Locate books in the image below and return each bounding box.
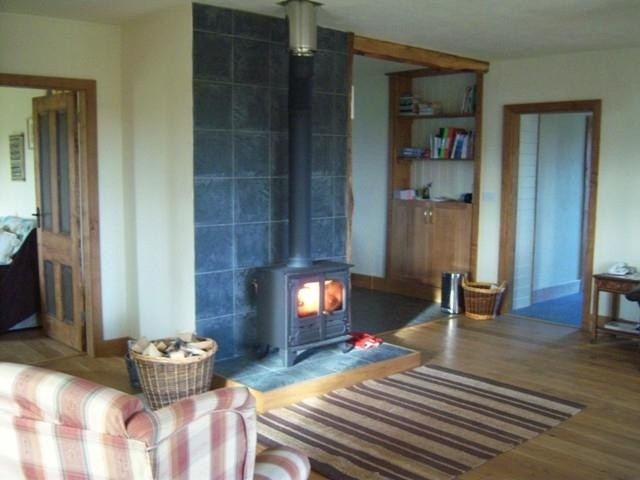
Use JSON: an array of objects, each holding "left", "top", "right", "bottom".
[{"left": 394, "top": 83, "right": 476, "bottom": 205}]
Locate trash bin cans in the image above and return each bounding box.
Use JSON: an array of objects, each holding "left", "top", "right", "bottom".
[{"left": 441, "top": 272, "right": 464, "bottom": 314}]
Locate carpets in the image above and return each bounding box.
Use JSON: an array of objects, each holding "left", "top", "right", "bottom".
[{"left": 257, "top": 364, "right": 587, "bottom": 480}]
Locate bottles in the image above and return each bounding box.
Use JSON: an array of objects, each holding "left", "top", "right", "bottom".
[{"left": 422, "top": 187, "right": 430, "bottom": 199}]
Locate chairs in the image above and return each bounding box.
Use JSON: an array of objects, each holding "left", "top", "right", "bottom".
[
  {"left": 0, "top": 361, "right": 311, "bottom": 480},
  {"left": 0, "top": 216, "right": 42, "bottom": 334}
]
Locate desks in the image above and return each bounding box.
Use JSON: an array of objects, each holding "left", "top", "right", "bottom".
[{"left": 591, "top": 273, "right": 640, "bottom": 343}]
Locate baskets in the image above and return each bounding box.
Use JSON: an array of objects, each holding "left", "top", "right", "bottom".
[
  {"left": 461, "top": 282, "right": 505, "bottom": 320},
  {"left": 129, "top": 337, "right": 217, "bottom": 411}
]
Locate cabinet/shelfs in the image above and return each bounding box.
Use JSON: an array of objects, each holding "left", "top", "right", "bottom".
[{"left": 384, "top": 68, "right": 484, "bottom": 303}]
